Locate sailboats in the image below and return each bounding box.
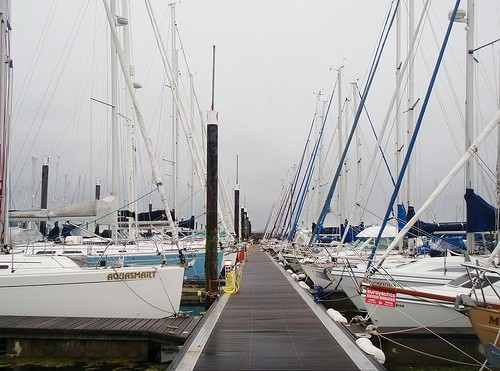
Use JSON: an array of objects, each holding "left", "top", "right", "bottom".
[
  {"left": 260, "top": 0, "right": 500, "bottom": 371},
  {"left": 0, "top": 0, "right": 248, "bottom": 319}
]
